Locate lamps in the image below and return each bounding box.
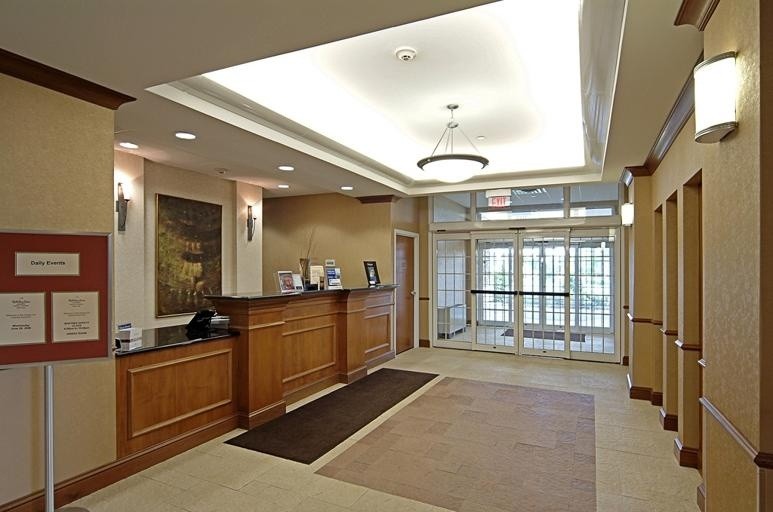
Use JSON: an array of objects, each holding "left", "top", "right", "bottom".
[
  {"left": 412, "top": 100, "right": 491, "bottom": 186},
  {"left": 619, "top": 202, "right": 634, "bottom": 228},
  {"left": 246, "top": 204, "right": 256, "bottom": 241},
  {"left": 691, "top": 49, "right": 737, "bottom": 144},
  {"left": 114, "top": 181, "right": 128, "bottom": 232}
]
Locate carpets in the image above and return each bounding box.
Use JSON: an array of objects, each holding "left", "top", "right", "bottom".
[
  {"left": 312, "top": 375, "right": 598, "bottom": 512},
  {"left": 219, "top": 364, "right": 442, "bottom": 467},
  {"left": 500, "top": 328, "right": 585, "bottom": 343}
]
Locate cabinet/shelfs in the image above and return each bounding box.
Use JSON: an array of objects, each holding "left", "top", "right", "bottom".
[{"left": 438, "top": 304, "right": 466, "bottom": 339}]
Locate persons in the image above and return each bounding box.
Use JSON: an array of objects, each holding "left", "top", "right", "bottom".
[{"left": 175, "top": 236, "right": 206, "bottom": 282}]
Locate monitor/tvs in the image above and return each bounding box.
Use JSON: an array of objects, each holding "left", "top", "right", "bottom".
[{"left": 304, "top": 283, "right": 318, "bottom": 291}]
